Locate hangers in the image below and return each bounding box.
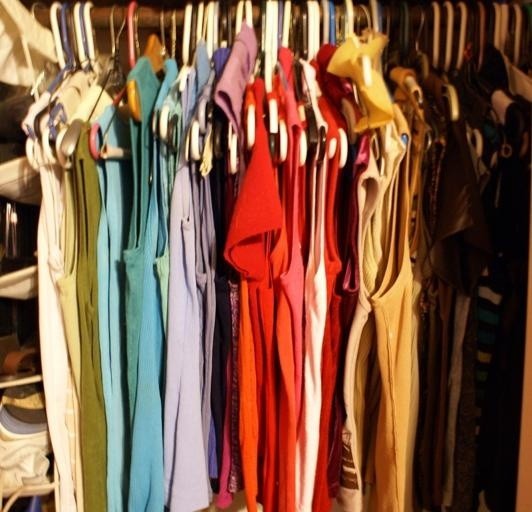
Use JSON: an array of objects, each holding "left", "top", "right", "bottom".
[{"left": 20, "top": 2, "right": 532, "bottom": 174}]
